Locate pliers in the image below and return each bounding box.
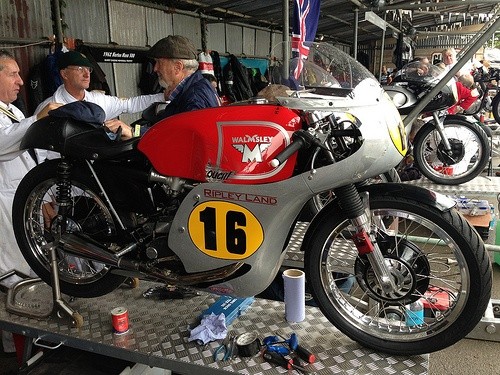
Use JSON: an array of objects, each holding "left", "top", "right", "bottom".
[{"left": 214, "top": 337, "right": 230, "bottom": 361}]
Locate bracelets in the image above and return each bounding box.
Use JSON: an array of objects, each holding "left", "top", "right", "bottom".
[{"left": 131, "top": 124, "right": 140, "bottom": 138}]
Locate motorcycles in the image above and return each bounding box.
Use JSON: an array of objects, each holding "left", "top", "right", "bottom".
[
  {"left": 376, "top": 54, "right": 500, "bottom": 185},
  {"left": 10, "top": 39, "right": 494, "bottom": 357}
]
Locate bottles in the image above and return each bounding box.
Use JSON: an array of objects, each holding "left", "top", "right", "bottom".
[
  {"left": 489, "top": 207, "right": 495, "bottom": 231},
  {"left": 405, "top": 300, "right": 424, "bottom": 326}
]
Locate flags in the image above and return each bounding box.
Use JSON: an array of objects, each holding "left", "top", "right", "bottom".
[{"left": 290, "top": 0, "right": 321, "bottom": 80}]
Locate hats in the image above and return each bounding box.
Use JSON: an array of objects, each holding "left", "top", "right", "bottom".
[
  {"left": 148, "top": 34, "right": 198, "bottom": 60},
  {"left": 56, "top": 51, "right": 94, "bottom": 69},
  {"left": 49, "top": 101, "right": 106, "bottom": 125}
]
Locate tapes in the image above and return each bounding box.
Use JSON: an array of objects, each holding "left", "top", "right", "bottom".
[{"left": 236, "top": 331, "right": 262, "bottom": 358}]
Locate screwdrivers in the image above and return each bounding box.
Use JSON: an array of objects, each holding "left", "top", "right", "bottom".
[
  {"left": 263, "top": 350, "right": 316, "bottom": 374},
  {"left": 275, "top": 331, "right": 316, "bottom": 364}
]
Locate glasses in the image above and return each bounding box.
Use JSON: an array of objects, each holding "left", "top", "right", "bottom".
[{"left": 67, "top": 66, "right": 93, "bottom": 73}]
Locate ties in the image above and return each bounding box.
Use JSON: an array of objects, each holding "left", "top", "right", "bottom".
[{"left": 6, "top": 110, "right": 39, "bottom": 165}]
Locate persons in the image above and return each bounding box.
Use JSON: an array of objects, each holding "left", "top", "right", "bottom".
[
  {"left": 381, "top": 47, "right": 490, "bottom": 176},
  {"left": 0, "top": 35, "right": 223, "bottom": 364}
]
